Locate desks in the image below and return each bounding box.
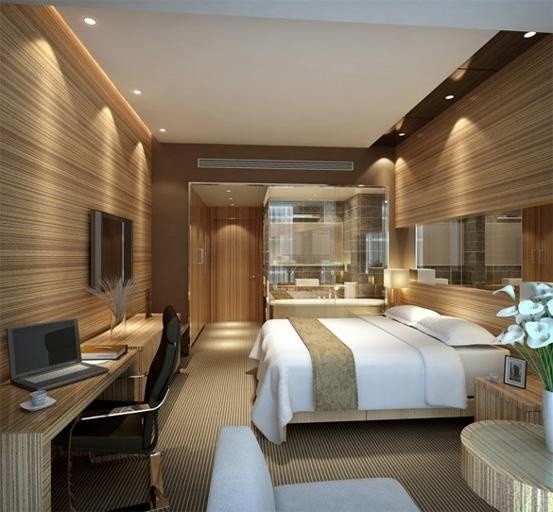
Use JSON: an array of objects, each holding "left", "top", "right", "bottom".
[
  {"left": 1, "top": 348, "right": 140, "bottom": 512},
  {"left": 459, "top": 419, "right": 553, "bottom": 511}
]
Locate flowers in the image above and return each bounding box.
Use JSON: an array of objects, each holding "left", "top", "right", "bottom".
[{"left": 491, "top": 284, "right": 553, "bottom": 393}]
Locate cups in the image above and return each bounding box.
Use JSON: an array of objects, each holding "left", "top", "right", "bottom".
[
  {"left": 30, "top": 389, "right": 48, "bottom": 406},
  {"left": 488, "top": 373, "right": 500, "bottom": 384}
]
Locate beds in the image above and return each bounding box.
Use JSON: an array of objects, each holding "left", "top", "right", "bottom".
[{"left": 247, "top": 314, "right": 512, "bottom": 446}]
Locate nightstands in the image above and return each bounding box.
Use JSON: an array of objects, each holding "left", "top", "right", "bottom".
[{"left": 472, "top": 376, "right": 542, "bottom": 422}]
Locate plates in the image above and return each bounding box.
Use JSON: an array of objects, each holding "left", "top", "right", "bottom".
[{"left": 19, "top": 396, "right": 56, "bottom": 411}]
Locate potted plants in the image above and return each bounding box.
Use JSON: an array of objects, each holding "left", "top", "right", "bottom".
[{"left": 85, "top": 275, "right": 140, "bottom": 339}]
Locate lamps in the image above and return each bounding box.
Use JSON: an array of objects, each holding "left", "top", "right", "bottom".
[
  {"left": 416, "top": 269, "right": 436, "bottom": 287},
  {"left": 384, "top": 268, "right": 409, "bottom": 305}
]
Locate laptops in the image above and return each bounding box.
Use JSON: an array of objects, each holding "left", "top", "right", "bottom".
[{"left": 7, "top": 319, "right": 108, "bottom": 392}]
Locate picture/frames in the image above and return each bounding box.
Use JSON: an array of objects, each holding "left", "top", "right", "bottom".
[{"left": 504, "top": 355, "right": 527, "bottom": 389}]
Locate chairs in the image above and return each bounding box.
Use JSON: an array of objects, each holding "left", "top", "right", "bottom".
[{"left": 54, "top": 304, "right": 182, "bottom": 512}]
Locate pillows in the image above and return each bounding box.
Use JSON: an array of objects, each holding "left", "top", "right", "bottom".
[
  {"left": 383, "top": 304, "right": 441, "bottom": 327},
  {"left": 414, "top": 315, "right": 499, "bottom": 347}
]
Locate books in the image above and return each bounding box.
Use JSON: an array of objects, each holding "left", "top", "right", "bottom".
[{"left": 79, "top": 343, "right": 128, "bottom": 361}]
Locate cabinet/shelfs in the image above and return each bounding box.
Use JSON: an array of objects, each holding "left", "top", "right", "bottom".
[{"left": 80, "top": 313, "right": 182, "bottom": 401}]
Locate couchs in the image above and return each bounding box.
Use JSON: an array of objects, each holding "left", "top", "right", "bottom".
[{"left": 205, "top": 424, "right": 420, "bottom": 511}]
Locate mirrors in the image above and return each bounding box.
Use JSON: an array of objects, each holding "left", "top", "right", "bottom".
[{"left": 415, "top": 208, "right": 523, "bottom": 296}]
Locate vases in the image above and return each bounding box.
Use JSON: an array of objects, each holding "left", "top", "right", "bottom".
[{"left": 542, "top": 389, "right": 553, "bottom": 453}]
